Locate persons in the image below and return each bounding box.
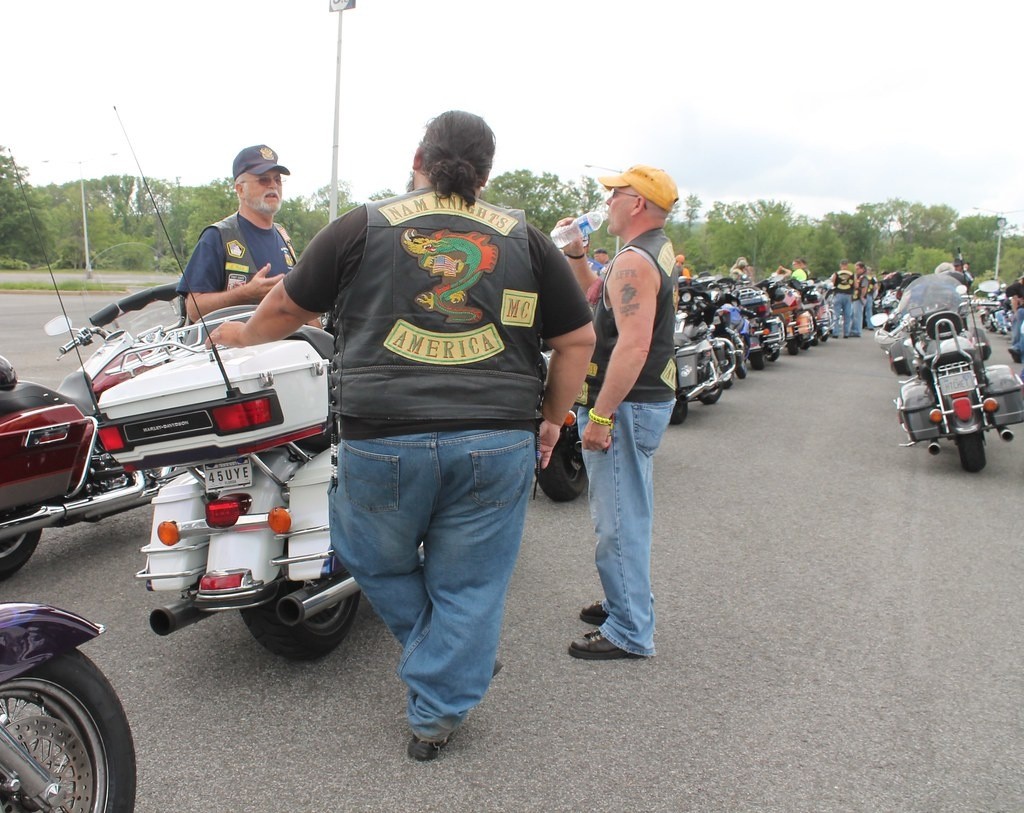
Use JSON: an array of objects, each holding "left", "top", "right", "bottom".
[
  {"left": 555, "top": 164, "right": 679, "bottom": 661},
  {"left": 205, "top": 110, "right": 596, "bottom": 762},
  {"left": 771, "top": 259, "right": 810, "bottom": 281},
  {"left": 593, "top": 249, "right": 611, "bottom": 264},
  {"left": 584, "top": 234, "right": 604, "bottom": 272},
  {"left": 988, "top": 296, "right": 1012, "bottom": 335},
  {"left": 865, "top": 267, "right": 878, "bottom": 331},
  {"left": 954, "top": 258, "right": 974, "bottom": 287},
  {"left": 849, "top": 263, "right": 868, "bottom": 337},
  {"left": 1005, "top": 276, "right": 1024, "bottom": 363},
  {"left": 175, "top": 145, "right": 322, "bottom": 329},
  {"left": 831, "top": 259, "right": 855, "bottom": 338},
  {"left": 729, "top": 256, "right": 753, "bottom": 280},
  {"left": 676, "top": 254, "right": 685, "bottom": 275}
]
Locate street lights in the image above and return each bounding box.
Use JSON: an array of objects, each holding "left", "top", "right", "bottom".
[
  {"left": 583, "top": 162, "right": 619, "bottom": 254},
  {"left": 970, "top": 206, "right": 1024, "bottom": 280}
]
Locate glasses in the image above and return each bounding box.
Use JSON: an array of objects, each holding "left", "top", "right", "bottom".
[
  {"left": 240, "top": 175, "right": 285, "bottom": 186},
  {"left": 612, "top": 189, "right": 648, "bottom": 209}
]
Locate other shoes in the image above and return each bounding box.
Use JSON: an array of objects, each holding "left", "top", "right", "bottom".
[
  {"left": 408, "top": 731, "right": 455, "bottom": 758},
  {"left": 1008, "top": 347, "right": 1021, "bottom": 363},
  {"left": 492, "top": 660, "right": 503, "bottom": 676}
]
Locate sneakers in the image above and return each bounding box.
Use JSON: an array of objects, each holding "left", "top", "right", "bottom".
[
  {"left": 569, "top": 630, "right": 645, "bottom": 659},
  {"left": 579, "top": 600, "right": 610, "bottom": 625}
]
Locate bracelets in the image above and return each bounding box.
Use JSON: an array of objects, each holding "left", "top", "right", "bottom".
[
  {"left": 567, "top": 253, "right": 584, "bottom": 259},
  {"left": 588, "top": 408, "right": 612, "bottom": 426}
]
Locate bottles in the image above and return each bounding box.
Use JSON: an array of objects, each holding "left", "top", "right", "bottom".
[{"left": 551, "top": 209, "right": 608, "bottom": 248}]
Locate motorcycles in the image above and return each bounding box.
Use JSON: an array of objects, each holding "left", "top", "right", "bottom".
[
  {"left": 531, "top": 270, "right": 1012, "bottom": 503},
  {"left": 92, "top": 304, "right": 425, "bottom": 662},
  {"left": 0, "top": 242, "right": 188, "bottom": 586},
  {"left": 870, "top": 272, "right": 1024, "bottom": 474},
  {"left": 0, "top": 599, "right": 139, "bottom": 813}
]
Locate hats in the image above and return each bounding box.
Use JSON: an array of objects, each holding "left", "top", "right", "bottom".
[
  {"left": 841, "top": 259, "right": 848, "bottom": 264},
  {"left": 739, "top": 260, "right": 748, "bottom": 267},
  {"left": 955, "top": 261, "right": 961, "bottom": 265},
  {"left": 233, "top": 145, "right": 290, "bottom": 180},
  {"left": 934, "top": 262, "right": 955, "bottom": 274},
  {"left": 598, "top": 164, "right": 679, "bottom": 212},
  {"left": 594, "top": 248, "right": 607, "bottom": 253}
]
